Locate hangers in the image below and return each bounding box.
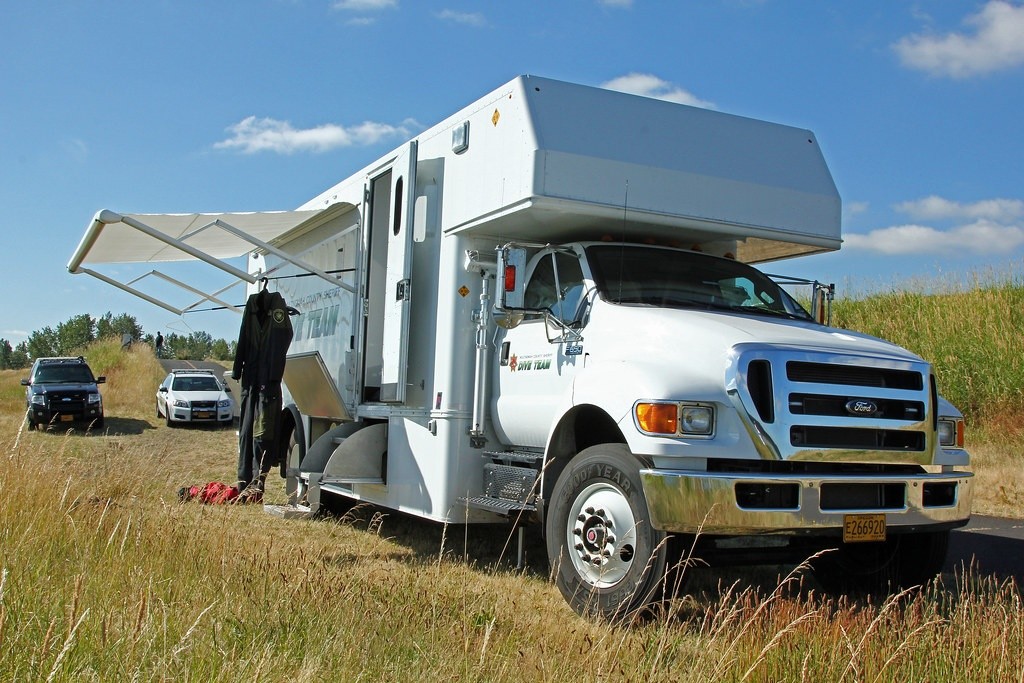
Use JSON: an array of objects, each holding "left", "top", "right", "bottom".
[{"left": 257, "top": 277, "right": 268, "bottom": 293}]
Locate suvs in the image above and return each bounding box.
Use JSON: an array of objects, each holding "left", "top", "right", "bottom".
[{"left": 19, "top": 355, "right": 107, "bottom": 430}]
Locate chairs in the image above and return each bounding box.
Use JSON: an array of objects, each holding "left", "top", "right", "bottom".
[{"left": 175, "top": 381, "right": 191, "bottom": 391}]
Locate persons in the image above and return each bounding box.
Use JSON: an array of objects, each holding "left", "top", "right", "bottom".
[{"left": 155, "top": 331, "right": 163, "bottom": 358}]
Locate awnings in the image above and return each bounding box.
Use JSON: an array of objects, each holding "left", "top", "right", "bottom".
[{"left": 66, "top": 202, "right": 359, "bottom": 316}]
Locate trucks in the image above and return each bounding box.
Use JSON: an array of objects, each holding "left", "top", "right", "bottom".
[{"left": 66, "top": 74, "right": 977, "bottom": 631}]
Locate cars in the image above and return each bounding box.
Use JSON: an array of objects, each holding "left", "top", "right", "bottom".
[{"left": 155, "top": 369, "right": 234, "bottom": 428}]
